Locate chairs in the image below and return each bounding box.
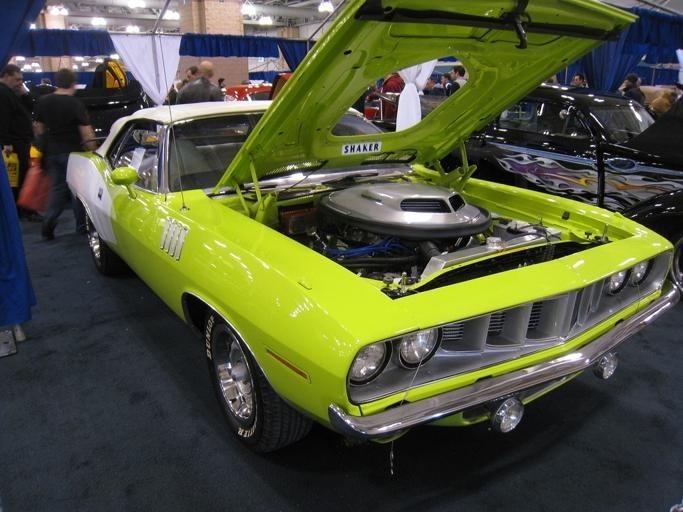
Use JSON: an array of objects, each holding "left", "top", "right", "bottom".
[{"left": 150, "top": 137, "right": 212, "bottom": 189}]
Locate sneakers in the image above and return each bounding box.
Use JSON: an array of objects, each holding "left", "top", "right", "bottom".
[
  {"left": 1, "top": 325, "right": 26, "bottom": 357},
  {"left": 29, "top": 214, "right": 55, "bottom": 239}
]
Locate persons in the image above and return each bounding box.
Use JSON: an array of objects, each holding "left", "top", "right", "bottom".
[
  {"left": 567, "top": 72, "right": 585, "bottom": 89},
  {"left": 617, "top": 72, "right": 646, "bottom": 107},
  {"left": 31, "top": 66, "right": 99, "bottom": 241},
  {"left": 0, "top": 64, "right": 46, "bottom": 224},
  {"left": 364, "top": 64, "right": 469, "bottom": 123},
  {"left": 641, "top": 86, "right": 678, "bottom": 120},
  {"left": 164, "top": 60, "right": 228, "bottom": 107}
]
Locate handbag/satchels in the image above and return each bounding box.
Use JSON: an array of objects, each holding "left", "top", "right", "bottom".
[{"left": 19, "top": 167, "right": 52, "bottom": 212}]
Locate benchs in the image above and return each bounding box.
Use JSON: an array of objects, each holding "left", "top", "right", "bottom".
[{"left": 137, "top": 142, "right": 244, "bottom": 191}]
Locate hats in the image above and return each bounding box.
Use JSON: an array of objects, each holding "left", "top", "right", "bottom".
[{"left": 626, "top": 74, "right": 637, "bottom": 83}]
